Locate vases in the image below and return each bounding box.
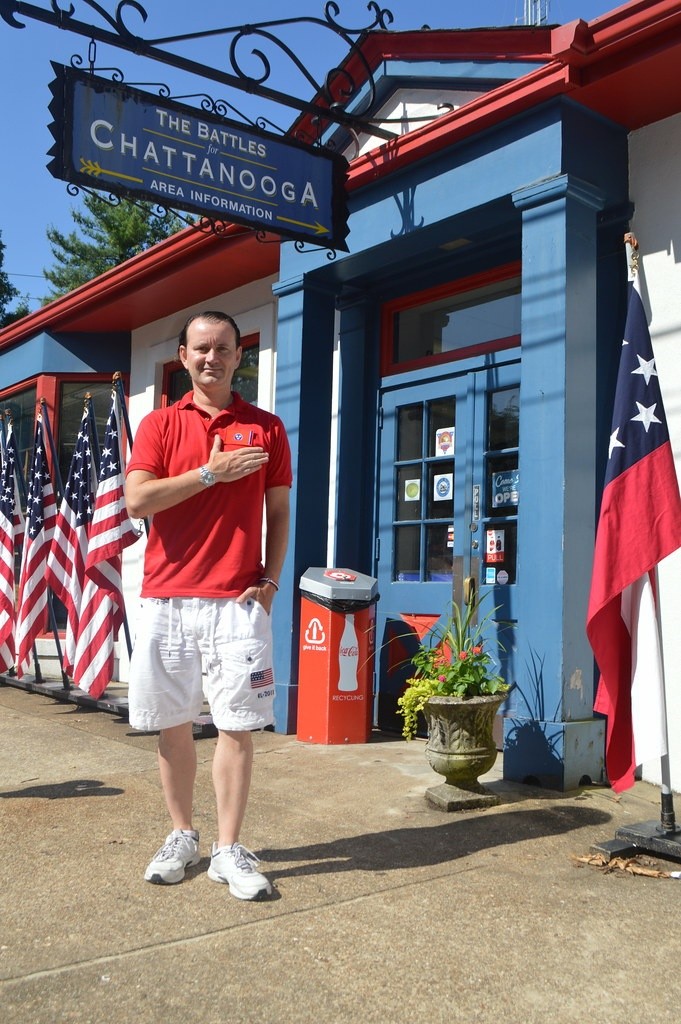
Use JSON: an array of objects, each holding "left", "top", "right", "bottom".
[{"left": 420, "top": 689, "right": 510, "bottom": 814}]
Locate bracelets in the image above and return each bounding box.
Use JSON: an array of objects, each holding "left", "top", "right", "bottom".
[{"left": 261, "top": 578, "right": 279, "bottom": 591}]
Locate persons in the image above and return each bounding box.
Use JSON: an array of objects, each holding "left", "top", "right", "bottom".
[{"left": 124, "top": 313, "right": 293, "bottom": 899}]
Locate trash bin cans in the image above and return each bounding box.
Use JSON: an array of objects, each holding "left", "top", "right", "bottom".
[{"left": 295, "top": 567, "right": 380, "bottom": 746}]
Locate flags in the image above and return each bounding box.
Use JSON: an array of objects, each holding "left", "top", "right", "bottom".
[
  {"left": 44, "top": 402, "right": 98, "bottom": 676},
  {"left": 0, "top": 419, "right": 25, "bottom": 673},
  {"left": 15, "top": 409, "right": 51, "bottom": 679},
  {"left": 587, "top": 268, "right": 681, "bottom": 794},
  {"left": 72, "top": 390, "right": 140, "bottom": 699}
]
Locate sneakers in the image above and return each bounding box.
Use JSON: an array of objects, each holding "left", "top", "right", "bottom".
[
  {"left": 144, "top": 829, "right": 201, "bottom": 885},
  {"left": 207, "top": 841, "right": 272, "bottom": 901}
]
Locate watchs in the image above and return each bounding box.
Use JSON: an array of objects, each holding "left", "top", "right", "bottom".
[{"left": 199, "top": 466, "right": 216, "bottom": 488}]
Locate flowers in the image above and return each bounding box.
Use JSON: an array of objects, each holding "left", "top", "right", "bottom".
[{"left": 350, "top": 588, "right": 521, "bottom": 741}]
